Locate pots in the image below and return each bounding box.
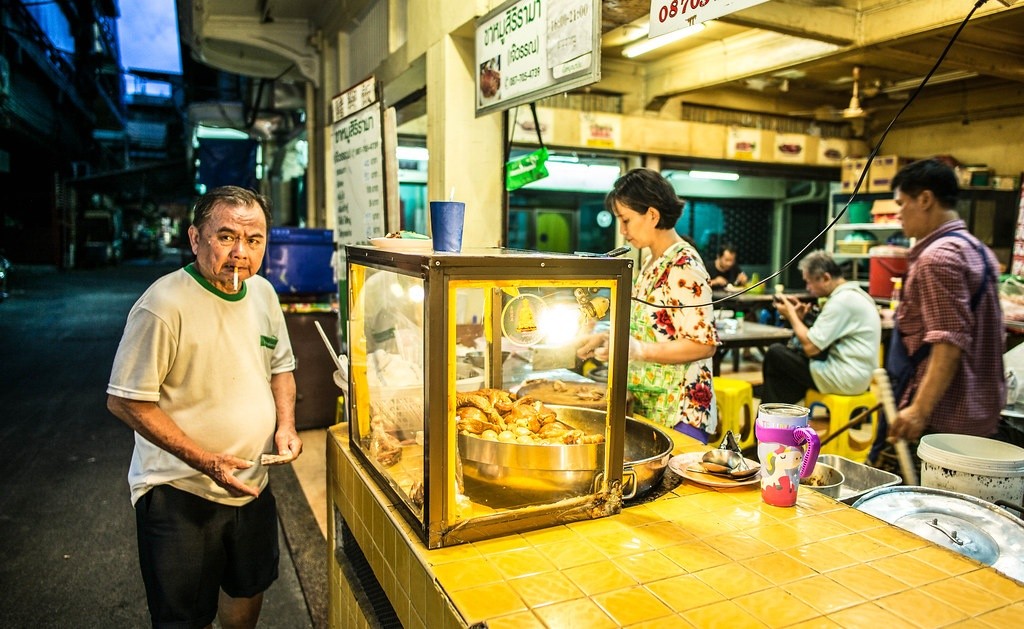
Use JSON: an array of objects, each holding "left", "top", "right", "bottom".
[
  {"left": 851, "top": 485, "right": 1024, "bottom": 583},
  {"left": 456, "top": 403, "right": 675, "bottom": 512}
]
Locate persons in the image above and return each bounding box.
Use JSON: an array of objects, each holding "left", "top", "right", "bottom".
[
  {"left": 575, "top": 168, "right": 722, "bottom": 445},
  {"left": 681, "top": 234, "right": 694, "bottom": 250},
  {"left": 704, "top": 243, "right": 761, "bottom": 364},
  {"left": 108, "top": 185, "right": 303, "bottom": 629},
  {"left": 869, "top": 159, "right": 1007, "bottom": 467},
  {"left": 762, "top": 249, "right": 881, "bottom": 404}
]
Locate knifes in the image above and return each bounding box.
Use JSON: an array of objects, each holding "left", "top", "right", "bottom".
[{"left": 533, "top": 346, "right": 578, "bottom": 371}]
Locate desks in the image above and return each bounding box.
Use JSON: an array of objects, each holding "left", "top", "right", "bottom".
[
  {"left": 712, "top": 285, "right": 820, "bottom": 368},
  {"left": 714, "top": 313, "right": 794, "bottom": 376}
]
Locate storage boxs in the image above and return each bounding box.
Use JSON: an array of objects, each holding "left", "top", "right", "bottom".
[
  {"left": 867, "top": 157, "right": 915, "bottom": 193},
  {"left": 838, "top": 157, "right": 868, "bottom": 194},
  {"left": 871, "top": 198, "right": 903, "bottom": 223}
]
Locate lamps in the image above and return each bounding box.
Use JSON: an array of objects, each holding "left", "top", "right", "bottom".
[{"left": 619, "top": 17, "right": 709, "bottom": 62}]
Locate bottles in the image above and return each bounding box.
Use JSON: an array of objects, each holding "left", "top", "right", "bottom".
[
  {"left": 890, "top": 281, "right": 902, "bottom": 310},
  {"left": 736, "top": 312, "right": 744, "bottom": 329}
]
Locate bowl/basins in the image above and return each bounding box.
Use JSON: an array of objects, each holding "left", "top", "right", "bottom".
[
  {"left": 800, "top": 461, "right": 846, "bottom": 498},
  {"left": 879, "top": 309, "right": 896, "bottom": 322},
  {"left": 465, "top": 351, "right": 511, "bottom": 369},
  {"left": 713, "top": 309, "right": 734, "bottom": 328}
]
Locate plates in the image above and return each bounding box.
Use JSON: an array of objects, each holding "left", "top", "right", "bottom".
[
  {"left": 521, "top": 121, "right": 548, "bottom": 134},
  {"left": 367, "top": 236, "right": 432, "bottom": 247},
  {"left": 734, "top": 140, "right": 843, "bottom": 161},
  {"left": 668, "top": 450, "right": 763, "bottom": 487},
  {"left": 480, "top": 71, "right": 500, "bottom": 105},
  {"left": 724, "top": 287, "right": 744, "bottom": 293}
]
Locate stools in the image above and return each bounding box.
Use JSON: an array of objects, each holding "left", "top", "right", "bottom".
[
  {"left": 712, "top": 376, "right": 754, "bottom": 450},
  {"left": 808, "top": 389, "right": 883, "bottom": 463}
]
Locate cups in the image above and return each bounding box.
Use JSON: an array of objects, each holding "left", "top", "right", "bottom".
[
  {"left": 723, "top": 319, "right": 738, "bottom": 335},
  {"left": 429, "top": 201, "right": 466, "bottom": 253},
  {"left": 754, "top": 402, "right": 821, "bottom": 508}
]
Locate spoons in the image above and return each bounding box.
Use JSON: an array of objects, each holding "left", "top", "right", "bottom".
[{"left": 686, "top": 467, "right": 762, "bottom": 481}]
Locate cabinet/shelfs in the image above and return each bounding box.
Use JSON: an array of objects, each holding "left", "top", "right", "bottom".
[{"left": 825, "top": 186, "right": 918, "bottom": 290}]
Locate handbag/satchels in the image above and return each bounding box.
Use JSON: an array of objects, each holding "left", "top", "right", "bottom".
[{"left": 786, "top": 312, "right": 829, "bottom": 361}]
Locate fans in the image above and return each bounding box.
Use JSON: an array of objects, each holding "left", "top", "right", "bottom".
[{"left": 791, "top": 63, "right": 904, "bottom": 130}]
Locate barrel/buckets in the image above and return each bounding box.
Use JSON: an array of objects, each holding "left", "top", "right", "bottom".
[
  {"left": 918, "top": 432, "right": 1024, "bottom": 518},
  {"left": 869, "top": 246, "right": 910, "bottom": 297}
]
